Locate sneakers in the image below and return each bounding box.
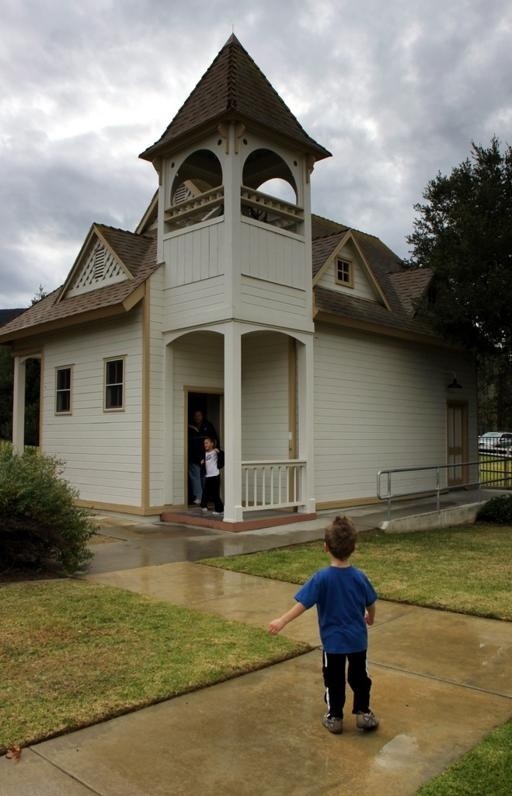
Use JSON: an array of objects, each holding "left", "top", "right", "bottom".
[
  {"left": 212, "top": 511, "right": 224, "bottom": 514},
  {"left": 201, "top": 506, "right": 208, "bottom": 511},
  {"left": 322, "top": 712, "right": 343, "bottom": 733},
  {"left": 356, "top": 712, "right": 379, "bottom": 727},
  {"left": 193, "top": 498, "right": 201, "bottom": 504}
]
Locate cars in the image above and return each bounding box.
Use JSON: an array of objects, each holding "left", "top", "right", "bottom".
[{"left": 478, "top": 432, "right": 512, "bottom": 448}]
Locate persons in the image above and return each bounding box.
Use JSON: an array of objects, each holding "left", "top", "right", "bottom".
[
  {"left": 268, "top": 515, "right": 380, "bottom": 734},
  {"left": 199, "top": 436, "right": 224, "bottom": 515},
  {"left": 189, "top": 408, "right": 218, "bottom": 504}
]
